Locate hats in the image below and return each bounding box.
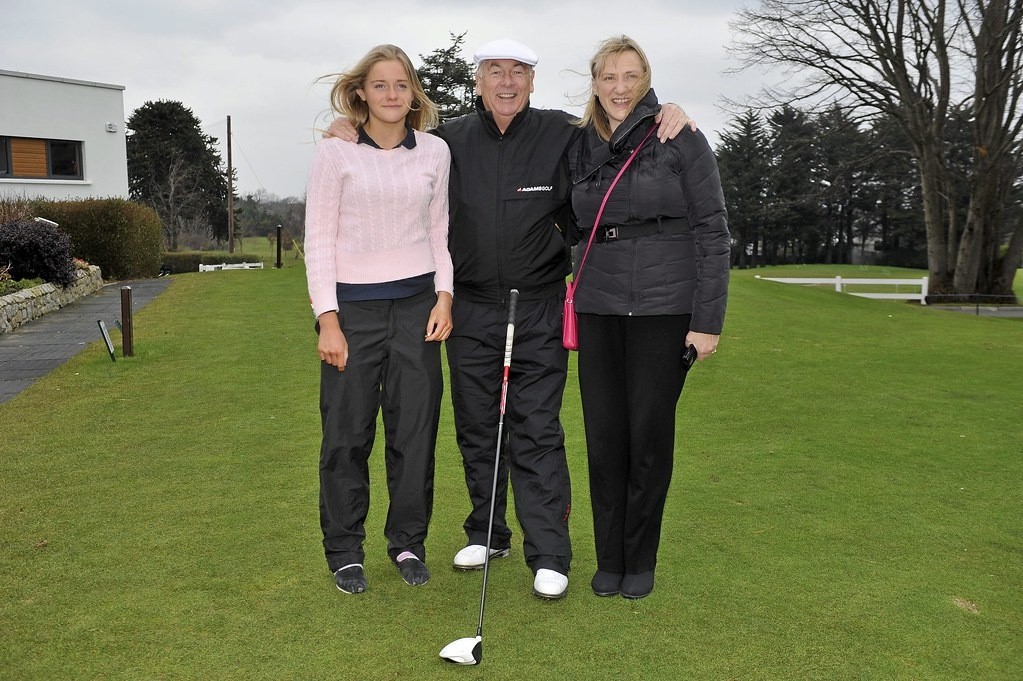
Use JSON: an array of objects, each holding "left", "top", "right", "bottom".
[{"left": 474, "top": 40, "right": 538, "bottom": 66}]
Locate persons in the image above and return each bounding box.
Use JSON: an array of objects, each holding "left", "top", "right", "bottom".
[
  {"left": 319, "top": 37, "right": 699, "bottom": 602},
  {"left": 565, "top": 36, "right": 730, "bottom": 598},
  {"left": 306, "top": 41, "right": 456, "bottom": 595}
]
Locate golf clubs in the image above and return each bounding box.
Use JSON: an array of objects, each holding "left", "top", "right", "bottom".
[{"left": 437, "top": 289, "right": 521, "bottom": 666}]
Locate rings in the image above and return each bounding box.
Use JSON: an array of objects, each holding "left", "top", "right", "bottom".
[{"left": 712, "top": 349, "right": 716, "bottom": 354}]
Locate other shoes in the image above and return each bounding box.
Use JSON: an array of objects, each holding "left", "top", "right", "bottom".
[
  {"left": 454, "top": 545, "right": 511, "bottom": 571},
  {"left": 592, "top": 570, "right": 655, "bottom": 598},
  {"left": 534, "top": 568, "right": 568, "bottom": 598},
  {"left": 334, "top": 567, "right": 366, "bottom": 594},
  {"left": 393, "top": 552, "right": 431, "bottom": 586}
]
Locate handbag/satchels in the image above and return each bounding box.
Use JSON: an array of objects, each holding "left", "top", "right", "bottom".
[{"left": 563, "top": 280, "right": 579, "bottom": 351}]
